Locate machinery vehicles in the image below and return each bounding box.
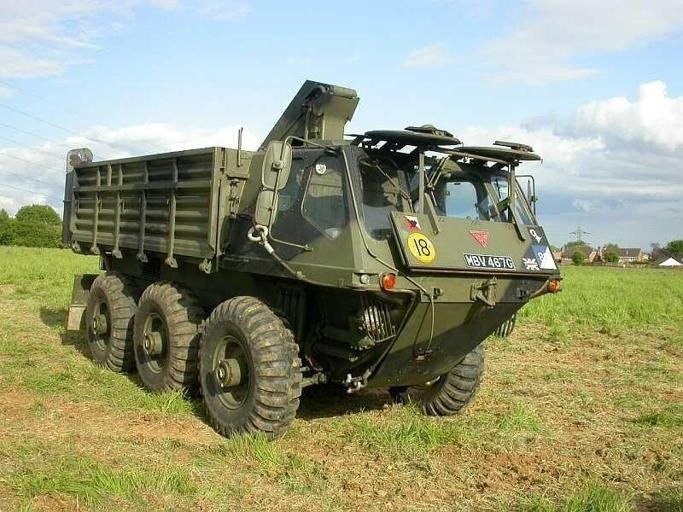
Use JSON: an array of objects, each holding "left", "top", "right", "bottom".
[{"left": 63, "top": 80, "right": 563, "bottom": 443}]
